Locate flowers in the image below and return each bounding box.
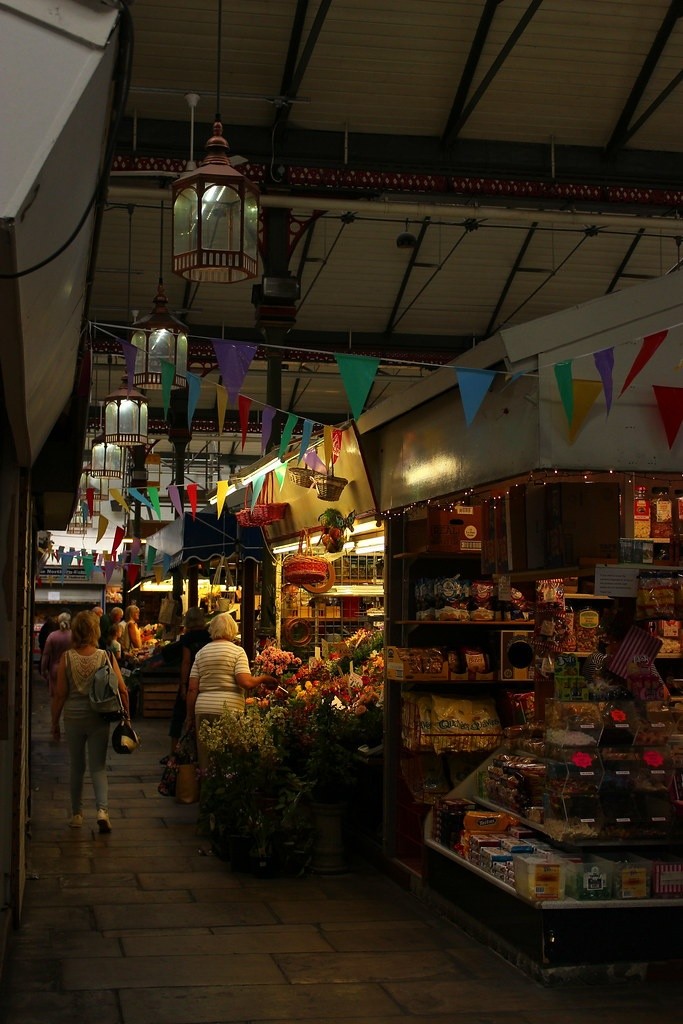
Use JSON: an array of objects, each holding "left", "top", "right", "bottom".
[{"left": 195, "top": 628, "right": 386, "bottom": 866}]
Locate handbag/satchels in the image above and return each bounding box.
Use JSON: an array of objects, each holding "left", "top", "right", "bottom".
[
  {"left": 157, "top": 733, "right": 200, "bottom": 805},
  {"left": 111, "top": 718, "right": 140, "bottom": 755},
  {"left": 158, "top": 591, "right": 176, "bottom": 625},
  {"left": 88, "top": 649, "right": 122, "bottom": 713}
]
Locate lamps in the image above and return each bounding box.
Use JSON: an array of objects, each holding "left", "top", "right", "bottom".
[
  {"left": 261, "top": 275, "right": 301, "bottom": 301},
  {"left": 272, "top": 520, "right": 385, "bottom": 556},
  {"left": 398, "top": 220, "right": 416, "bottom": 248},
  {"left": 140, "top": 579, "right": 238, "bottom": 592},
  {"left": 210, "top": 448, "right": 304, "bottom": 505},
  {"left": 80, "top": 355, "right": 112, "bottom": 502},
  {"left": 131, "top": 201, "right": 189, "bottom": 390},
  {"left": 67, "top": 464, "right": 109, "bottom": 534},
  {"left": 131, "top": 471, "right": 147, "bottom": 480},
  {"left": 307, "top": 584, "right": 384, "bottom": 597},
  {"left": 170, "top": 0, "right": 261, "bottom": 284},
  {"left": 91, "top": 354, "right": 127, "bottom": 480},
  {"left": 104, "top": 203, "right": 148, "bottom": 446}
]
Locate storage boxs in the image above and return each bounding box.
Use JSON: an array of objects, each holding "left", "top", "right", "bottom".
[
  {"left": 433, "top": 797, "right": 478, "bottom": 847},
  {"left": 546, "top": 483, "right": 621, "bottom": 566},
  {"left": 500, "top": 629, "right": 535, "bottom": 680},
  {"left": 409, "top": 507, "right": 482, "bottom": 553},
  {"left": 546, "top": 698, "right": 683, "bottom": 844},
  {"left": 513, "top": 852, "right": 683, "bottom": 902}
]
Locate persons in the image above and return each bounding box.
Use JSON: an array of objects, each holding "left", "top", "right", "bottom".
[
  {"left": 38, "top": 616, "right": 60, "bottom": 673},
  {"left": 40, "top": 613, "right": 73, "bottom": 697},
  {"left": 92, "top": 606, "right": 142, "bottom": 719},
  {"left": 582, "top": 604, "right": 668, "bottom": 695},
  {"left": 50, "top": 609, "right": 130, "bottom": 833},
  {"left": 168, "top": 605, "right": 212, "bottom": 751},
  {"left": 184, "top": 613, "right": 278, "bottom": 800}
]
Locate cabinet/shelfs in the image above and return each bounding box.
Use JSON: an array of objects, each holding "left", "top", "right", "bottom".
[{"left": 391, "top": 473, "right": 682, "bottom": 870}]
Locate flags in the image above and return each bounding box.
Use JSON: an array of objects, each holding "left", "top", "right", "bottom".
[{"left": 608, "top": 625, "right": 663, "bottom": 679}]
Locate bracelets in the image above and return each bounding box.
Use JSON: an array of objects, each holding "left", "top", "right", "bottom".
[
  {"left": 124, "top": 719, "right": 130, "bottom": 721},
  {"left": 185, "top": 720, "right": 194, "bottom": 724}
]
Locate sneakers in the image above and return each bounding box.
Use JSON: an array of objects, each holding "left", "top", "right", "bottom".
[
  {"left": 96, "top": 809, "right": 113, "bottom": 834},
  {"left": 66, "top": 813, "right": 83, "bottom": 829}
]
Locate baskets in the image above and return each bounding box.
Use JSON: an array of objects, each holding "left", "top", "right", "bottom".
[
  {"left": 286, "top": 446, "right": 325, "bottom": 489},
  {"left": 314, "top": 452, "right": 348, "bottom": 502},
  {"left": 233, "top": 482, "right": 261, "bottom": 528},
  {"left": 282, "top": 528, "right": 328, "bottom": 584},
  {"left": 252, "top": 471, "right": 288, "bottom": 525}
]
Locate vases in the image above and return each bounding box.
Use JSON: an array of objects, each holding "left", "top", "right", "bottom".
[
  {"left": 305, "top": 804, "right": 360, "bottom": 881},
  {"left": 248, "top": 840, "right": 300, "bottom": 879},
  {"left": 227, "top": 834, "right": 251, "bottom": 870},
  {"left": 256, "top": 796, "right": 280, "bottom": 822}
]
